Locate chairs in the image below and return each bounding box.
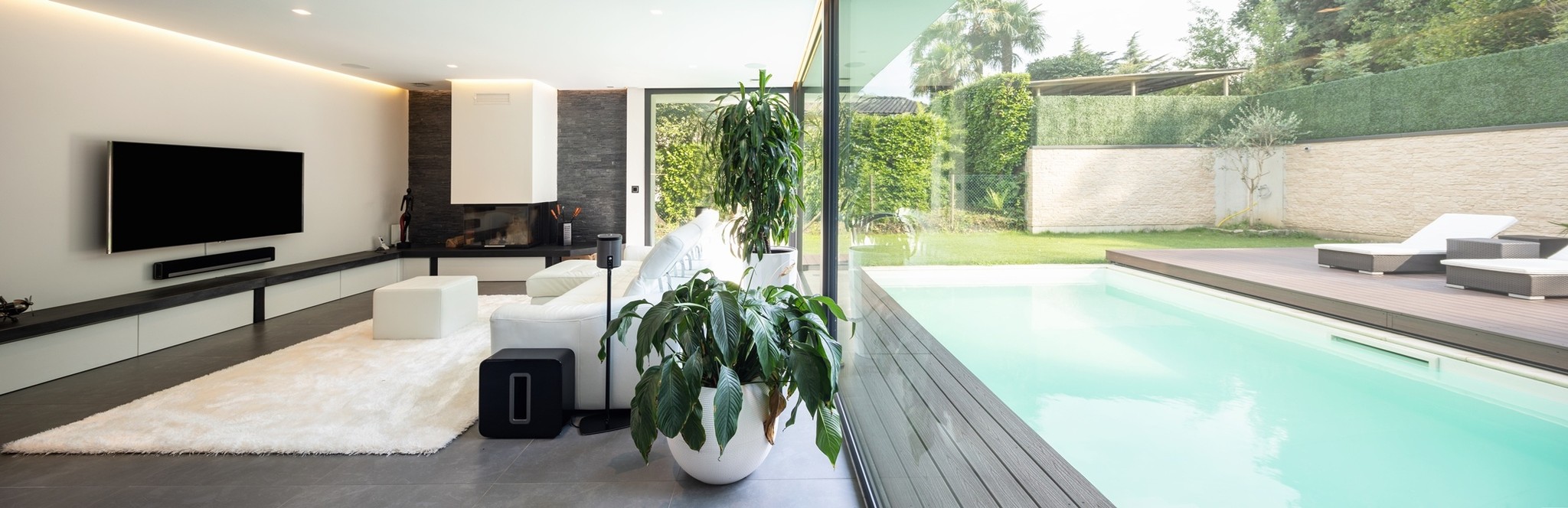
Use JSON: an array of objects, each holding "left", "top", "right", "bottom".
[
  {"left": 1438, "top": 243, "right": 1568, "bottom": 301},
  {"left": 1312, "top": 212, "right": 1519, "bottom": 275}
]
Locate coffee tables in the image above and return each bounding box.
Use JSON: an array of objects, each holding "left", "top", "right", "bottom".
[{"left": 370, "top": 274, "right": 479, "bottom": 341}]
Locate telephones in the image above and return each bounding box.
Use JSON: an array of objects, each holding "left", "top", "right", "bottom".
[{"left": 377, "top": 236, "right": 389, "bottom": 250}]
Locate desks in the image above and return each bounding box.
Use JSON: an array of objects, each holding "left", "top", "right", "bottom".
[{"left": 1444, "top": 238, "right": 1541, "bottom": 261}]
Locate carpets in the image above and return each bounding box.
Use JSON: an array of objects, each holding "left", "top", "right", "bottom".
[{"left": 0, "top": 293, "right": 529, "bottom": 453}]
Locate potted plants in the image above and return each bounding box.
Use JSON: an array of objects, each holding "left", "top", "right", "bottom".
[
  {"left": 838, "top": 90, "right": 933, "bottom": 360},
  {"left": 693, "top": 64, "right": 814, "bottom": 291},
  {"left": 595, "top": 261, "right": 856, "bottom": 491}
]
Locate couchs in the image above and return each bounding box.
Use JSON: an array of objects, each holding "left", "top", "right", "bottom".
[{"left": 489, "top": 206, "right": 724, "bottom": 415}]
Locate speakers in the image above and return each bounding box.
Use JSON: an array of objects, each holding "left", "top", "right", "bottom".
[{"left": 478, "top": 349, "right": 576, "bottom": 440}]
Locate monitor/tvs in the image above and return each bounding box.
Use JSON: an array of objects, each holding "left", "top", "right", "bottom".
[{"left": 105, "top": 141, "right": 304, "bottom": 254}]
